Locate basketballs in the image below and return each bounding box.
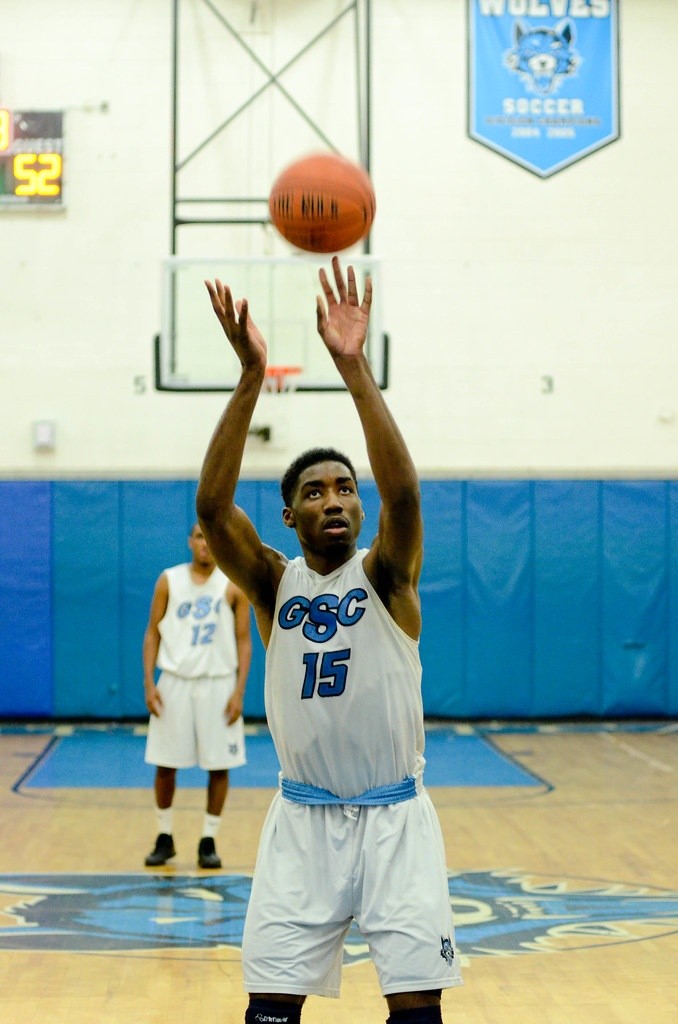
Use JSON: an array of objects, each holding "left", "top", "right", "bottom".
[{"left": 268, "top": 154, "right": 377, "bottom": 252}]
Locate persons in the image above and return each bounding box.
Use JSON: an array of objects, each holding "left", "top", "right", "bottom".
[
  {"left": 144, "top": 526, "right": 251, "bottom": 867},
  {"left": 195, "top": 257, "right": 462, "bottom": 1024}
]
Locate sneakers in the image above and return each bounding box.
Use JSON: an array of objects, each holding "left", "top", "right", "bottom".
[
  {"left": 146, "top": 833, "right": 177, "bottom": 868},
  {"left": 197, "top": 838, "right": 221, "bottom": 870}
]
